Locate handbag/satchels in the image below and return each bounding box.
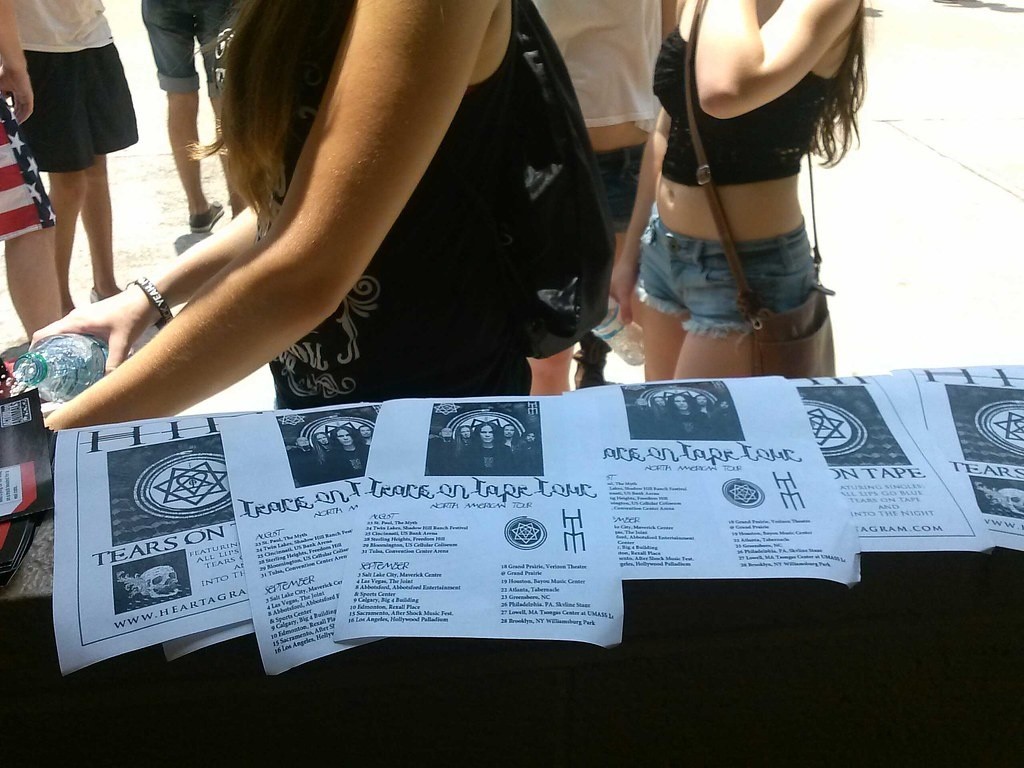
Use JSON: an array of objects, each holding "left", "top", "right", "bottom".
[{"left": 752, "top": 278, "right": 836, "bottom": 380}]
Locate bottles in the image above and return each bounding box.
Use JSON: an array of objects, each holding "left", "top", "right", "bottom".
[
  {"left": 590, "top": 294, "right": 645, "bottom": 367},
  {"left": 13, "top": 332, "right": 135, "bottom": 403}
]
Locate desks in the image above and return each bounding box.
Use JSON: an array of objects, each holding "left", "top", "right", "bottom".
[{"left": 0, "top": 368, "right": 1024, "bottom": 768}]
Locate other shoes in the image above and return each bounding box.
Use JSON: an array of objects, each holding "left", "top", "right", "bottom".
[{"left": 90, "top": 286, "right": 104, "bottom": 302}]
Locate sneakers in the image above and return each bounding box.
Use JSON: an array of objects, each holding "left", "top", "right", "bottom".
[{"left": 189, "top": 201, "right": 224, "bottom": 232}]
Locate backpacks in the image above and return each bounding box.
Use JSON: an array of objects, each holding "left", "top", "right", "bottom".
[{"left": 511, "top": 0, "right": 617, "bottom": 359}]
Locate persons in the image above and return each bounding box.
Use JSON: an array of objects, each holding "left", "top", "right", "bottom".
[
  {"left": 426, "top": 422, "right": 542, "bottom": 476},
  {"left": 0, "top": 0, "right": 64, "bottom": 343},
  {"left": 141, "top": 0, "right": 253, "bottom": 234},
  {"left": 628, "top": 393, "right": 741, "bottom": 441},
  {"left": 30, "top": 1, "right": 542, "bottom": 434},
  {"left": 609, "top": 1, "right": 869, "bottom": 389},
  {"left": 287, "top": 426, "right": 373, "bottom": 487},
  {"left": 529, "top": 1, "right": 682, "bottom": 388},
  {"left": 8, "top": 0, "right": 138, "bottom": 318}
]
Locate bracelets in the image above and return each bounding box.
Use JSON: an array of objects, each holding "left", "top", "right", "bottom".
[{"left": 125, "top": 277, "right": 173, "bottom": 330}]
